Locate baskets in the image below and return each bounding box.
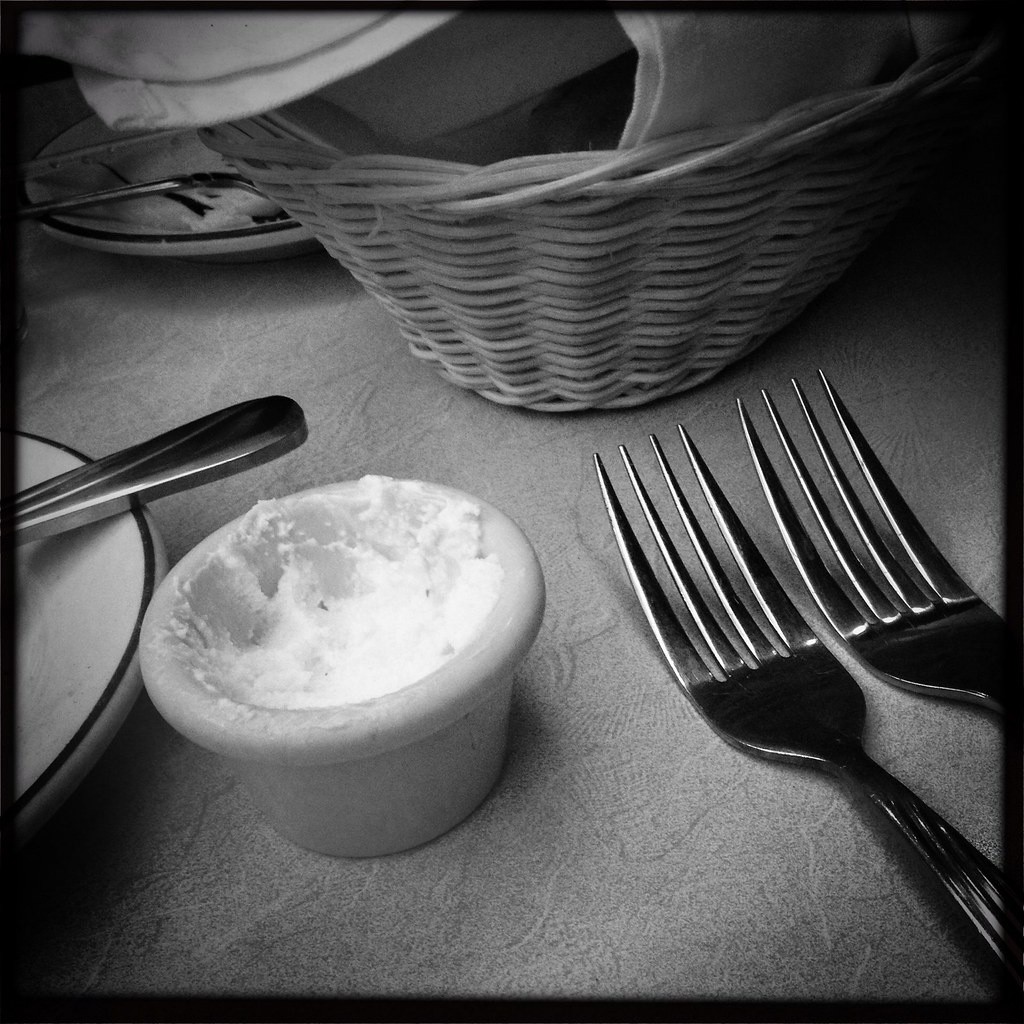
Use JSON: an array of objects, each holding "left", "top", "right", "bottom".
[{"left": 197, "top": 11, "right": 996, "bottom": 414}]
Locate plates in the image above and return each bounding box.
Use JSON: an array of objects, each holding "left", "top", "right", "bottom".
[
  {"left": 18, "top": 111, "right": 322, "bottom": 262},
  {"left": 18, "top": 431, "right": 170, "bottom": 859}
]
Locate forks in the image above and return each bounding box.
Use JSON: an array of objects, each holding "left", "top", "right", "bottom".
[
  {"left": 592, "top": 424, "right": 1005, "bottom": 962},
  {"left": 736, "top": 370, "right": 1002, "bottom": 712}
]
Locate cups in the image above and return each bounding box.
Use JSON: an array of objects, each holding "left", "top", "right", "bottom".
[{"left": 140, "top": 478, "right": 545, "bottom": 855}]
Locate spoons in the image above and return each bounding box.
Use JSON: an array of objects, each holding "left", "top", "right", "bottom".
[{"left": 19, "top": 172, "right": 270, "bottom": 221}]
